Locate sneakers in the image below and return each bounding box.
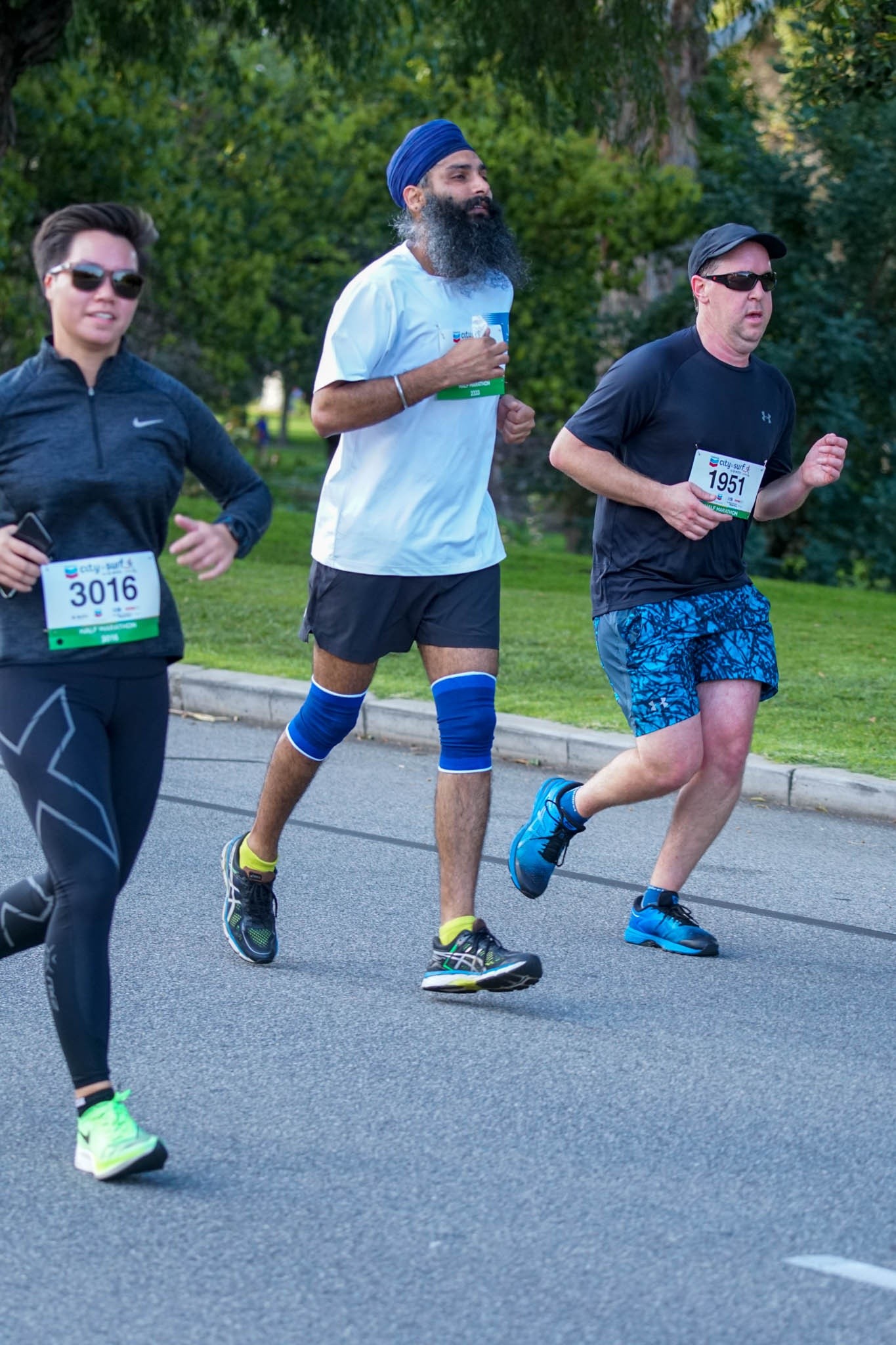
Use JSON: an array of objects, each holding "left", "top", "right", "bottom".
[
  {"left": 220, "top": 831, "right": 278, "bottom": 964},
  {"left": 507, "top": 776, "right": 587, "bottom": 899},
  {"left": 421, "top": 918, "right": 543, "bottom": 994},
  {"left": 624, "top": 896, "right": 719, "bottom": 956},
  {"left": 74, "top": 1088, "right": 168, "bottom": 1180}
]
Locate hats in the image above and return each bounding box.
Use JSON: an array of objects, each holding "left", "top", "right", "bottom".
[
  {"left": 386, "top": 118, "right": 475, "bottom": 210},
  {"left": 688, "top": 223, "right": 787, "bottom": 282}
]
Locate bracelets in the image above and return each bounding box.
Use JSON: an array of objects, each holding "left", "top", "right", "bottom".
[{"left": 394, "top": 374, "right": 408, "bottom": 411}]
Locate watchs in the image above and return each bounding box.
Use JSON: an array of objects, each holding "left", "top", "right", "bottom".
[{"left": 218, "top": 515, "right": 249, "bottom": 554}]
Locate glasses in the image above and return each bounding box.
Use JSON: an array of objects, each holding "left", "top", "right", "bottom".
[
  {"left": 46, "top": 259, "right": 146, "bottom": 300},
  {"left": 704, "top": 270, "right": 777, "bottom": 292}
]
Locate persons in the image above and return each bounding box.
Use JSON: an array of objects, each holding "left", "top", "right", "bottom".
[
  {"left": 508, "top": 223, "right": 849, "bottom": 959},
  {"left": 0, "top": 199, "right": 272, "bottom": 1182},
  {"left": 219, "top": 118, "right": 546, "bottom": 996}
]
